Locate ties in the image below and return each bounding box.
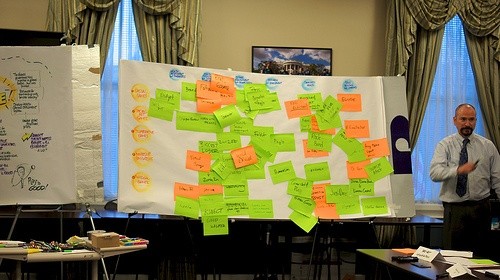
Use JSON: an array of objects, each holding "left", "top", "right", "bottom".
[{"left": 455, "top": 138, "right": 470, "bottom": 197}]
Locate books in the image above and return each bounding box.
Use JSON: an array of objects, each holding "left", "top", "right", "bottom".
[{"left": 460, "top": 259, "right": 500, "bottom": 269}]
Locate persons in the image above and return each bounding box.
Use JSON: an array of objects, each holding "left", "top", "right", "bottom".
[{"left": 429, "top": 104, "right": 500, "bottom": 258}]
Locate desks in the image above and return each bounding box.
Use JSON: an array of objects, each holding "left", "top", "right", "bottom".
[
  {"left": 0, "top": 205, "right": 445, "bottom": 275},
  {"left": 0, "top": 248, "right": 141, "bottom": 280},
  {"left": 355, "top": 249, "right": 450, "bottom": 280}
]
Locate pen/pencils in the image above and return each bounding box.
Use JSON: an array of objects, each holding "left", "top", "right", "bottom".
[{"left": 474, "top": 159, "right": 480, "bottom": 165}]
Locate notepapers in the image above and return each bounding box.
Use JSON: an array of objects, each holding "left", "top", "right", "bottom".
[{"left": 392, "top": 248, "right": 417, "bottom": 254}]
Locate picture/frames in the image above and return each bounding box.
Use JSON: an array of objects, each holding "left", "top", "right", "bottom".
[{"left": 251, "top": 46, "right": 332, "bottom": 76}]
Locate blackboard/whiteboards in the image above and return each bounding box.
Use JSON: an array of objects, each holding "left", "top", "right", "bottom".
[
  {"left": 117, "top": 58, "right": 417, "bottom": 220},
  {"left": 0, "top": 44, "right": 104, "bottom": 205}
]
